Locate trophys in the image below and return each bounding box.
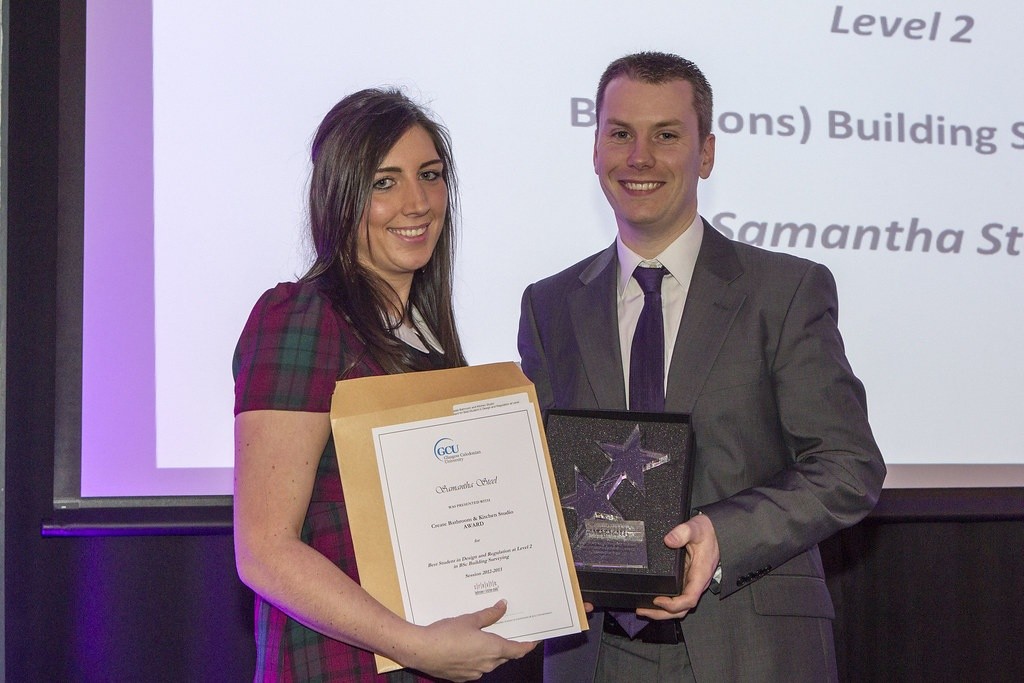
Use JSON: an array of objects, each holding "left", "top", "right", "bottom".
[{"left": 537, "top": 408, "right": 697, "bottom": 599}]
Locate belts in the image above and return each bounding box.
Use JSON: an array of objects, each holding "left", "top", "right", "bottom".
[{"left": 603, "top": 612, "right": 685, "bottom": 645}]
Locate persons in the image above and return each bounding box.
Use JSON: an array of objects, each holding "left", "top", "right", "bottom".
[
  {"left": 518, "top": 52, "right": 887, "bottom": 682},
  {"left": 232, "top": 89, "right": 539, "bottom": 683}
]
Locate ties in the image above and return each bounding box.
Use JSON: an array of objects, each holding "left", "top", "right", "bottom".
[{"left": 605, "top": 265, "right": 673, "bottom": 639}]
